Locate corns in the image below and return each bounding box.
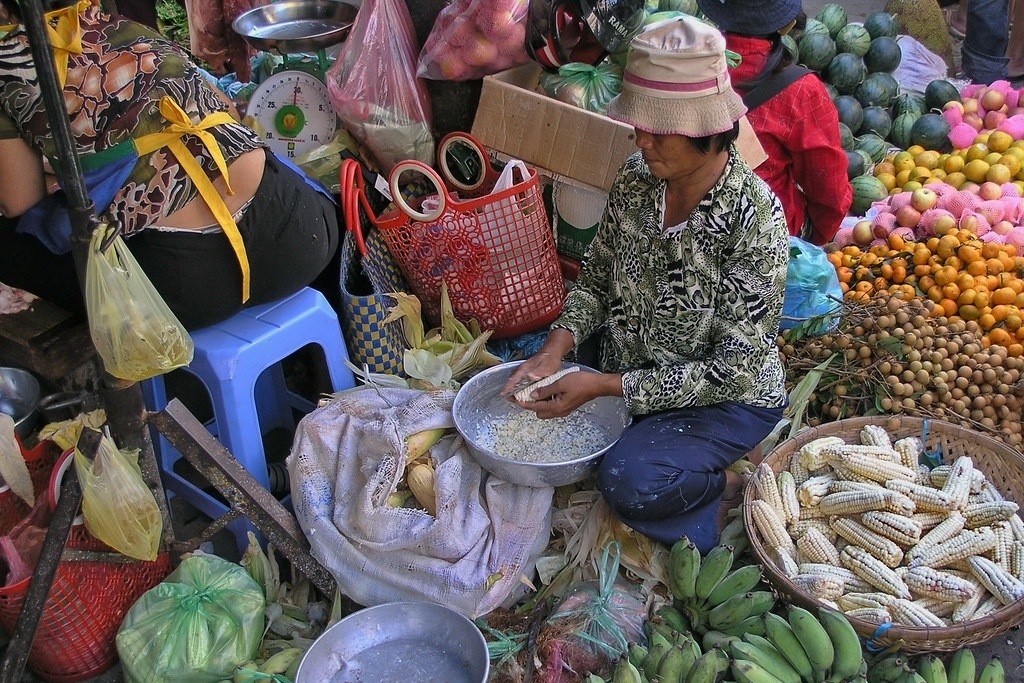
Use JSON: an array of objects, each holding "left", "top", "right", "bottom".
[
  {"left": 383, "top": 426, "right": 448, "bottom": 516},
  {"left": 116, "top": 557, "right": 265, "bottom": 683},
  {"left": 749, "top": 424, "right": 1024, "bottom": 626},
  {"left": 514, "top": 366, "right": 580, "bottom": 401}
]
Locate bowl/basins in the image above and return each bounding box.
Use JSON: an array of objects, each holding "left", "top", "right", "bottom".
[
  {"left": 0, "top": 367, "right": 41, "bottom": 440},
  {"left": 451, "top": 360, "right": 633, "bottom": 487}
]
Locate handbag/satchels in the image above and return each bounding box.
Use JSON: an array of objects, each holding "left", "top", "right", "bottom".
[
  {"left": 74, "top": 433, "right": 162, "bottom": 562},
  {"left": 85, "top": 222, "right": 194, "bottom": 379},
  {"left": 781, "top": 236, "right": 843, "bottom": 333},
  {"left": 374, "top": 128, "right": 566, "bottom": 339},
  {"left": 0, "top": 436, "right": 172, "bottom": 683},
  {"left": 114, "top": 553, "right": 266, "bottom": 683},
  {"left": 416, "top": 0, "right": 533, "bottom": 85},
  {"left": 325, "top": 0, "right": 436, "bottom": 178},
  {"left": 539, "top": 56, "right": 624, "bottom": 115},
  {"left": 337, "top": 156, "right": 410, "bottom": 378}
]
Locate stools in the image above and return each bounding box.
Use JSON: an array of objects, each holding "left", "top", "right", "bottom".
[{"left": 138, "top": 285, "right": 356, "bottom": 557}]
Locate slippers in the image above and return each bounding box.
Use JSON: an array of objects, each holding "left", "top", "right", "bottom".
[{"left": 943, "top": 9, "right": 968, "bottom": 40}]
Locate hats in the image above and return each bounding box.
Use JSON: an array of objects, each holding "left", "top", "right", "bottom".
[
  {"left": 695, "top": 0, "right": 802, "bottom": 35},
  {"left": 607, "top": 14, "right": 747, "bottom": 136}
]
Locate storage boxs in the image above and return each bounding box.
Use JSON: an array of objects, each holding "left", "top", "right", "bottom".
[{"left": 470, "top": 62, "right": 770, "bottom": 261}]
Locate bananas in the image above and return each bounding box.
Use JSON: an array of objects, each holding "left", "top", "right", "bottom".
[
  {"left": 582, "top": 532, "right": 1008, "bottom": 683},
  {"left": 218, "top": 647, "right": 307, "bottom": 683}
]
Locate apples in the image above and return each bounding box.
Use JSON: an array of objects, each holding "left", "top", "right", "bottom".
[
  {"left": 428, "top": 0, "right": 590, "bottom": 109},
  {"left": 941, "top": 88, "right": 1024, "bottom": 135},
  {"left": 833, "top": 181, "right": 1024, "bottom": 258}
]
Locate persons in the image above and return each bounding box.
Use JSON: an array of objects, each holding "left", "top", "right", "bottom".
[
  {"left": 937, "top": 0, "right": 1024, "bottom": 94},
  {"left": 0, "top": 0, "right": 352, "bottom": 322},
  {"left": 500, "top": 16, "right": 791, "bottom": 555},
  {"left": 697, "top": 0, "right": 853, "bottom": 250}
]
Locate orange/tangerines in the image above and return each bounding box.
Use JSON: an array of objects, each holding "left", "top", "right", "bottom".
[
  {"left": 824, "top": 224, "right": 1023, "bottom": 364},
  {"left": 872, "top": 131, "right": 1024, "bottom": 195}
]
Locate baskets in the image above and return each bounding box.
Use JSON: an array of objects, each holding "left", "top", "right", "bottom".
[{"left": 744, "top": 416, "right": 1024, "bottom": 656}]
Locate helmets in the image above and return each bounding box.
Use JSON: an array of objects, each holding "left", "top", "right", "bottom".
[{"left": 525, "top": 0, "right": 611, "bottom": 74}]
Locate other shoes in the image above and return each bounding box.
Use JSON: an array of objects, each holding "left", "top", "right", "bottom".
[
  {"left": 946, "top": 72, "right": 974, "bottom": 94},
  {"left": 713, "top": 473, "right": 745, "bottom": 535},
  {"left": 1004, "top": 69, "right": 1024, "bottom": 82}
]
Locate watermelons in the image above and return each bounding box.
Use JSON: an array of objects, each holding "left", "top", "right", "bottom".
[{"left": 637, "top": 0, "right": 960, "bottom": 215}]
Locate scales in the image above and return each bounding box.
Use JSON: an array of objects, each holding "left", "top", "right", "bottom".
[{"left": 231, "top": 0, "right": 361, "bottom": 164}]
